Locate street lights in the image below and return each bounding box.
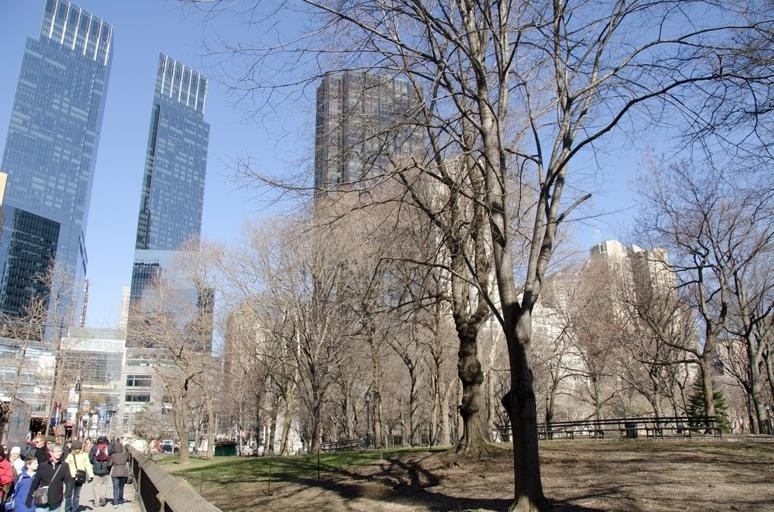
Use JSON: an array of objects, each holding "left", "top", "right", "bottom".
[
  {"left": 364, "top": 391, "right": 372, "bottom": 450},
  {"left": 73, "top": 380, "right": 83, "bottom": 443}
]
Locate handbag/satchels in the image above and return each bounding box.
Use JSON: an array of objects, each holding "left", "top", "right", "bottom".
[
  {"left": 75, "top": 470, "right": 86, "bottom": 484},
  {"left": 126, "top": 473, "right": 133, "bottom": 483},
  {"left": 33, "top": 486, "right": 49, "bottom": 505}
]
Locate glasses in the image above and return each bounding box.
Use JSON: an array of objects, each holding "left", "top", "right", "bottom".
[{"left": 47, "top": 443, "right": 52, "bottom": 445}]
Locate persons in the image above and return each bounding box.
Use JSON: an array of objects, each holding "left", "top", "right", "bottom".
[{"left": 0, "top": 436, "right": 129, "bottom": 512}]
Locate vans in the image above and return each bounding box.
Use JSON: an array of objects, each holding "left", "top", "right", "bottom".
[{"left": 160, "top": 440, "right": 174, "bottom": 448}]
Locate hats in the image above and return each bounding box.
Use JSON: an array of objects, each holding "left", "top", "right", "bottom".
[
  {"left": 71, "top": 442, "right": 82, "bottom": 450},
  {"left": 44, "top": 435, "right": 56, "bottom": 444},
  {"left": 10, "top": 446, "right": 21, "bottom": 454}
]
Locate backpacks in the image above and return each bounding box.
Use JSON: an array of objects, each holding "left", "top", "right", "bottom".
[{"left": 96, "top": 444, "right": 108, "bottom": 461}]
